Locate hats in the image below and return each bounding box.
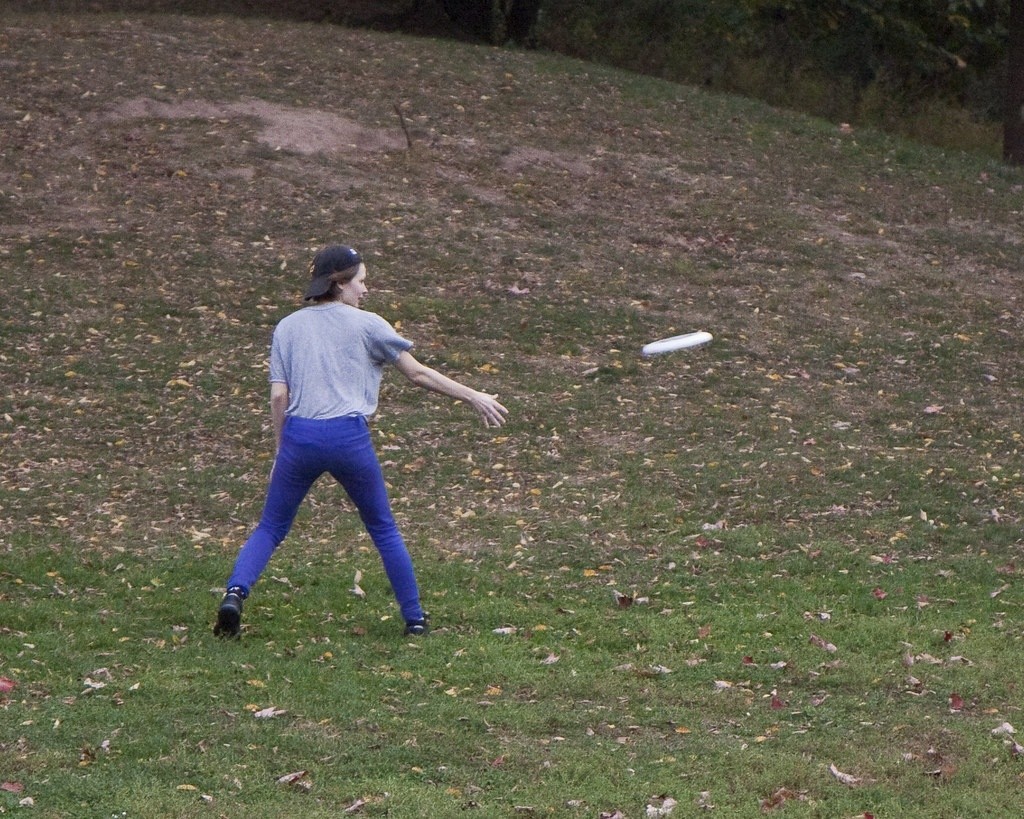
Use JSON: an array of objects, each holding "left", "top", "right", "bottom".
[{"left": 304, "top": 245, "right": 362, "bottom": 301}]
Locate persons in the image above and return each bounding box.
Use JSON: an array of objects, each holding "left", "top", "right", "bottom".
[{"left": 214, "top": 244, "right": 509, "bottom": 637}]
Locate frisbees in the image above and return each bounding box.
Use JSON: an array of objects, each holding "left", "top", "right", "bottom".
[{"left": 642, "top": 332, "right": 712, "bottom": 355}]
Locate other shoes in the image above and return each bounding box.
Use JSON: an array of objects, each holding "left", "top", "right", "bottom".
[
  {"left": 213, "top": 586, "right": 244, "bottom": 641},
  {"left": 405, "top": 619, "right": 427, "bottom": 637}
]
